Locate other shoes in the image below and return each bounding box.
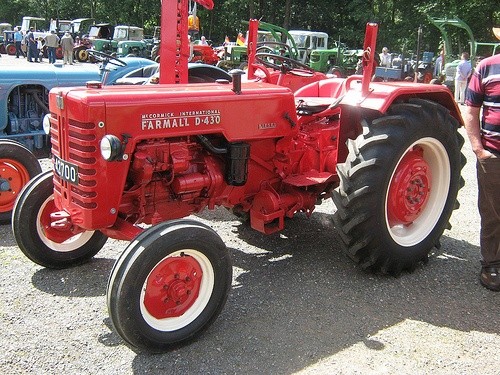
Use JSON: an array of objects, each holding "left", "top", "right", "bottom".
[{"left": 13, "top": 54, "right": 76, "bottom": 65}]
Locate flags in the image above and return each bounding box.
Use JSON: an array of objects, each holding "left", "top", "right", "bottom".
[
  {"left": 236, "top": 31, "right": 246, "bottom": 46},
  {"left": 225, "top": 35, "right": 230, "bottom": 45}
]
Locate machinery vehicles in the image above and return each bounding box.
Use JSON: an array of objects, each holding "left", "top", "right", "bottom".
[
  {"left": 0, "top": 8, "right": 500, "bottom": 101},
  {"left": 12, "top": 0, "right": 467, "bottom": 354},
  {"left": 0, "top": 49, "right": 247, "bottom": 225}
]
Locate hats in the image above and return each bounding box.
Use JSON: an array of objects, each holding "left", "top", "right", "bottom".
[
  {"left": 493, "top": 27, "right": 500, "bottom": 40},
  {"left": 63, "top": 33, "right": 71, "bottom": 38}
]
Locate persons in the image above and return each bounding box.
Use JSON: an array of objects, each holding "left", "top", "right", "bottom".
[
  {"left": 198, "top": 36, "right": 209, "bottom": 46},
  {"left": 13, "top": 26, "right": 76, "bottom": 65},
  {"left": 454, "top": 53, "right": 472, "bottom": 105},
  {"left": 379, "top": 47, "right": 391, "bottom": 67},
  {"left": 463, "top": 25, "right": 500, "bottom": 291}
]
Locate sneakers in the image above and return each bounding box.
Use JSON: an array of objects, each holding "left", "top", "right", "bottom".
[{"left": 480, "top": 266, "right": 500, "bottom": 292}]
